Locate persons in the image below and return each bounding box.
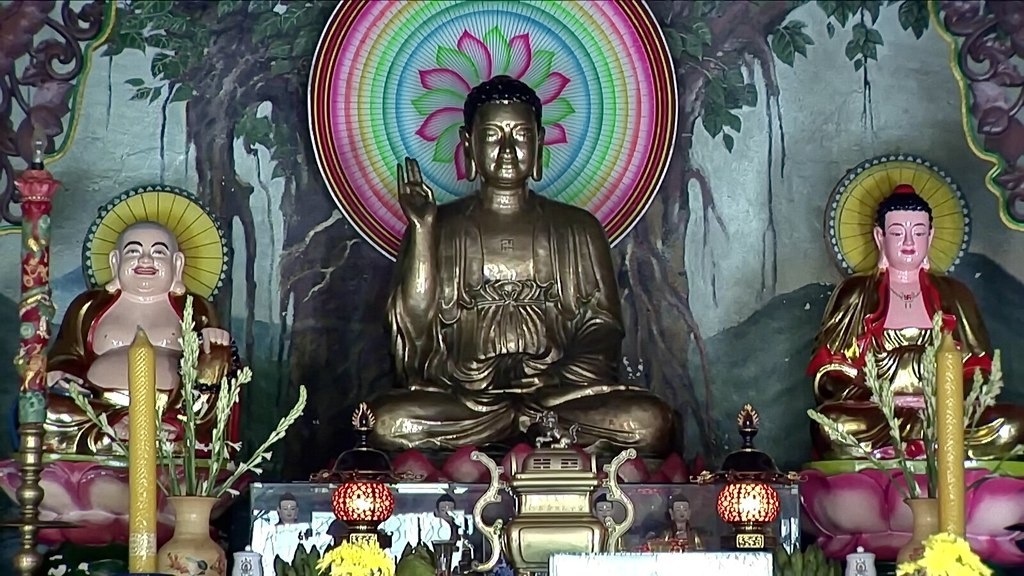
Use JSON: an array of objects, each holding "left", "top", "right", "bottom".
[
  {"left": 593, "top": 493, "right": 626, "bottom": 552},
  {"left": 44, "top": 221, "right": 235, "bottom": 462},
  {"left": 430, "top": 493, "right": 463, "bottom": 559},
  {"left": 263, "top": 492, "right": 309, "bottom": 575},
  {"left": 371, "top": 76, "right": 681, "bottom": 461},
  {"left": 809, "top": 184, "right": 995, "bottom": 459},
  {"left": 659, "top": 493, "right": 703, "bottom": 550}
]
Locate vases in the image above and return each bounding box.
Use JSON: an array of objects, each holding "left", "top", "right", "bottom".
[
  {"left": 158, "top": 498, "right": 227, "bottom": 576},
  {"left": 897, "top": 495, "right": 939, "bottom": 576}
]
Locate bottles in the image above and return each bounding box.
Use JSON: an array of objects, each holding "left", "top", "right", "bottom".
[
  {"left": 232, "top": 544, "right": 263, "bottom": 576},
  {"left": 846, "top": 545, "right": 878, "bottom": 576}
]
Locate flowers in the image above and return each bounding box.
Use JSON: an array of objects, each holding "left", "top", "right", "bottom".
[
  {"left": 314, "top": 540, "right": 394, "bottom": 576},
  {"left": 808, "top": 311, "right": 1005, "bottom": 499},
  {"left": 68, "top": 295, "right": 306, "bottom": 501},
  {"left": 895, "top": 529, "right": 996, "bottom": 576}
]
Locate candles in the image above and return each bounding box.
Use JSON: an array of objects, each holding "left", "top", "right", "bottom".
[
  {"left": 935, "top": 317, "right": 966, "bottom": 537},
  {"left": 130, "top": 327, "right": 154, "bottom": 574}
]
